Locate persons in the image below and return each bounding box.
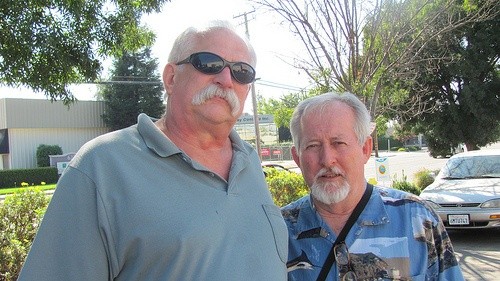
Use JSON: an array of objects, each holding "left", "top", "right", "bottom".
[
  {"left": 16, "top": 21, "right": 290, "bottom": 280},
  {"left": 280, "top": 92, "right": 466, "bottom": 281}
]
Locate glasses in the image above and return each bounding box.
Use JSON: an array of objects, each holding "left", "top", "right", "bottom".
[
  {"left": 175, "top": 52, "right": 256, "bottom": 84},
  {"left": 333, "top": 241, "right": 358, "bottom": 281}
]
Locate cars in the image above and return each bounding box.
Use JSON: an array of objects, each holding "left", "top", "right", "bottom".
[{"left": 419, "top": 149, "right": 500, "bottom": 236}]
